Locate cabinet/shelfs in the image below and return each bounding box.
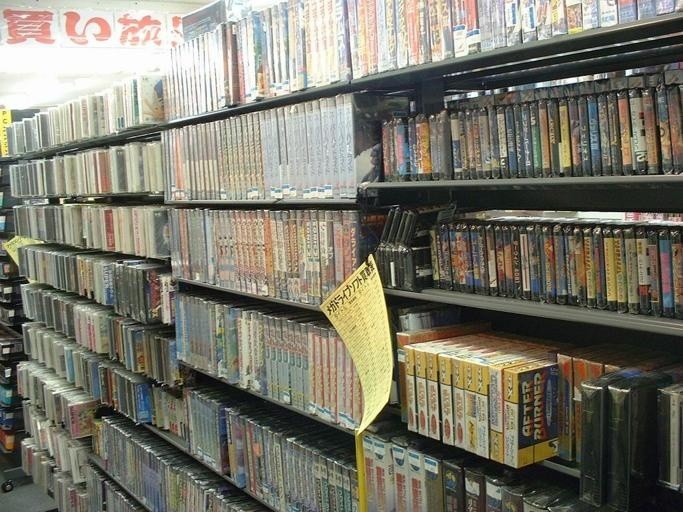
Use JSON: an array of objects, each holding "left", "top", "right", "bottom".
[{"left": 1, "top": 10, "right": 683, "bottom": 510}]
